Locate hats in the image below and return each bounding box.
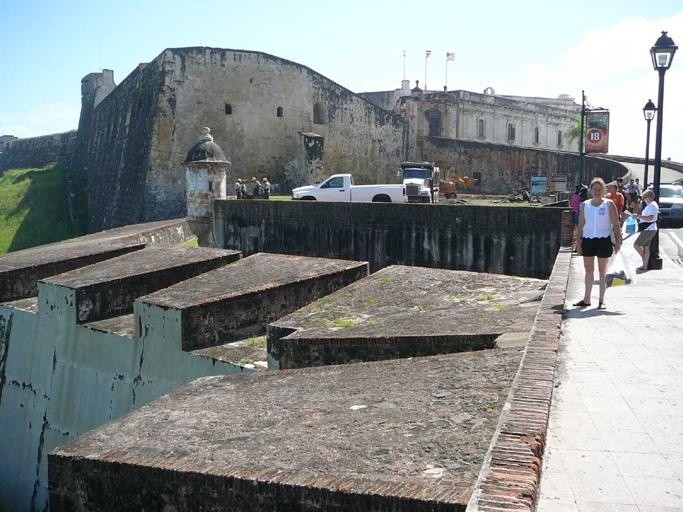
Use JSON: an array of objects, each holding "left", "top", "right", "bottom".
[
  {"left": 580, "top": 189, "right": 589, "bottom": 198},
  {"left": 576, "top": 185, "right": 587, "bottom": 192}
]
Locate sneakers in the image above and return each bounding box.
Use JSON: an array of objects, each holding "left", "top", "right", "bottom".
[{"left": 637, "top": 267, "right": 647, "bottom": 271}]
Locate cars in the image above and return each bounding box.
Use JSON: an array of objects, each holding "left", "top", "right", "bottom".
[{"left": 648, "top": 184, "right": 683, "bottom": 227}]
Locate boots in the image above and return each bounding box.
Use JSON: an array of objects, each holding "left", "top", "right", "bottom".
[{"left": 598, "top": 302, "right": 606, "bottom": 309}]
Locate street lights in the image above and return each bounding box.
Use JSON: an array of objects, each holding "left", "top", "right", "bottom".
[
  {"left": 642, "top": 100, "right": 658, "bottom": 211},
  {"left": 648, "top": 30, "right": 677, "bottom": 269}
]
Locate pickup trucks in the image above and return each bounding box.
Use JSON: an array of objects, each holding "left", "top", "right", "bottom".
[{"left": 292, "top": 174, "right": 409, "bottom": 203}]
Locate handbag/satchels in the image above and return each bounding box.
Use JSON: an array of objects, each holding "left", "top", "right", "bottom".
[{"left": 638, "top": 221, "right": 654, "bottom": 231}]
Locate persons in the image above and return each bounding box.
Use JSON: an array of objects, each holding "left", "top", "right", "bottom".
[
  {"left": 569, "top": 177, "right": 643, "bottom": 253},
  {"left": 634, "top": 188, "right": 659, "bottom": 271},
  {"left": 236, "top": 176, "right": 270, "bottom": 200},
  {"left": 571, "top": 176, "right": 622, "bottom": 310}
]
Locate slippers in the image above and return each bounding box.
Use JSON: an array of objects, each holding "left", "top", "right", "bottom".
[{"left": 573, "top": 301, "right": 591, "bottom": 306}]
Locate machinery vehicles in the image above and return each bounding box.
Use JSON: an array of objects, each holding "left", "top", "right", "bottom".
[
  {"left": 439, "top": 175, "right": 473, "bottom": 199},
  {"left": 401, "top": 160, "right": 440, "bottom": 204}
]
